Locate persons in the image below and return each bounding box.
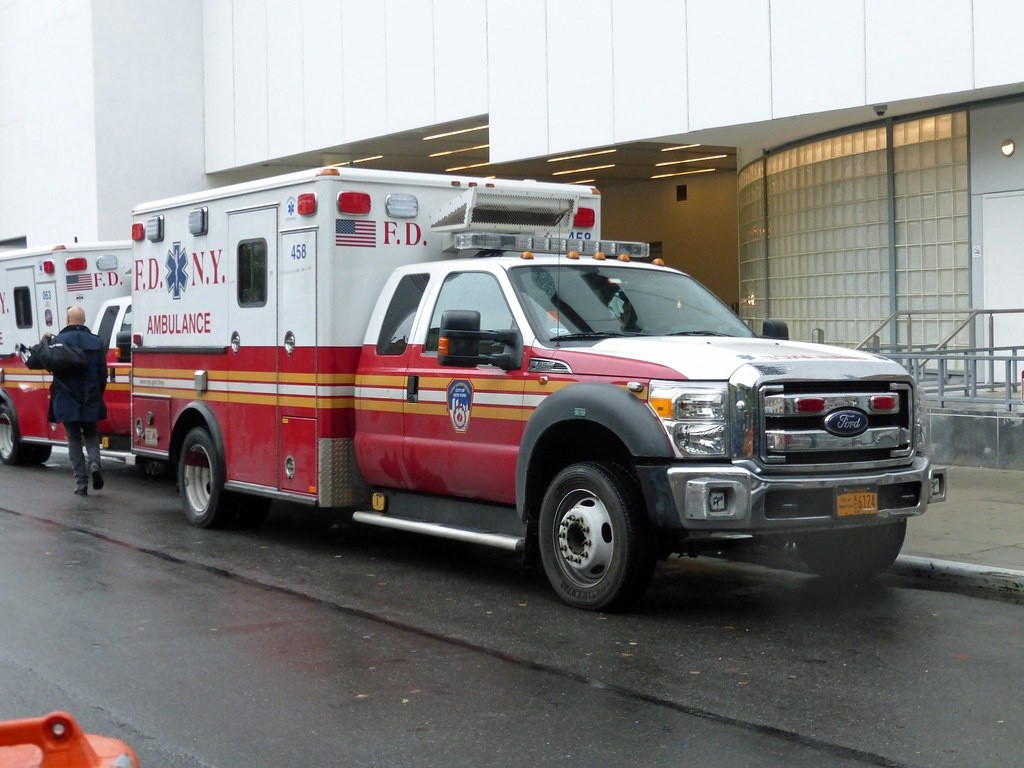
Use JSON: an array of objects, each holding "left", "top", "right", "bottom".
[
  {"left": 40, "top": 307, "right": 108, "bottom": 494},
  {"left": 619, "top": 291, "right": 641, "bottom": 332}
]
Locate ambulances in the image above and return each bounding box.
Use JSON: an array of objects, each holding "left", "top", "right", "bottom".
[
  {"left": 132, "top": 167, "right": 950, "bottom": 605},
  {"left": 0, "top": 238, "right": 134, "bottom": 465}
]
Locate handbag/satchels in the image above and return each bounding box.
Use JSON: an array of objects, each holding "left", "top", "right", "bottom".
[{"left": 16, "top": 335, "right": 88, "bottom": 372}]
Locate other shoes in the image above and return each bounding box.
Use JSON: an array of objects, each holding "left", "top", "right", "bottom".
[
  {"left": 73, "top": 483, "right": 88, "bottom": 495},
  {"left": 90, "top": 464, "right": 104, "bottom": 489}
]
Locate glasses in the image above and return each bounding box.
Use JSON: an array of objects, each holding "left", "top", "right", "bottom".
[{"left": 66, "top": 306, "right": 72, "bottom": 324}]
